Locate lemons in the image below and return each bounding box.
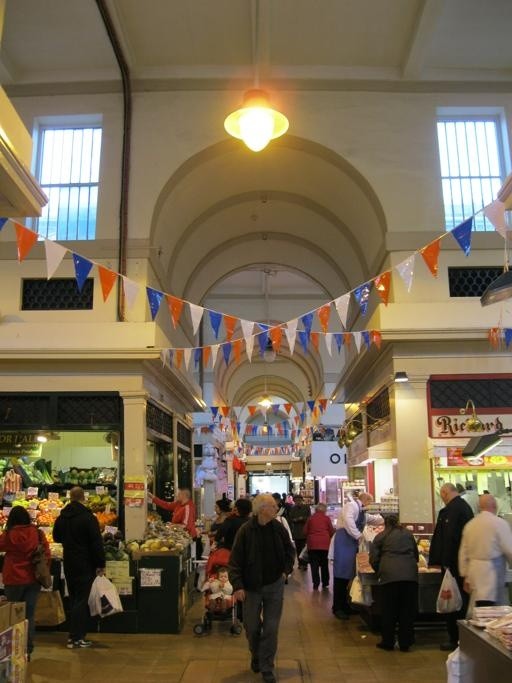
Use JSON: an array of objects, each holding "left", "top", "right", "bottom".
[{"left": 125, "top": 535, "right": 185, "bottom": 552}]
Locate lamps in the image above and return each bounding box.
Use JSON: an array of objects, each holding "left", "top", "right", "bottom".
[
  {"left": 480, "top": 270, "right": 512, "bottom": 307},
  {"left": 224, "top": 0, "right": 290, "bottom": 153},
  {"left": 461, "top": 429, "right": 511, "bottom": 460},
  {"left": 262, "top": 271, "right": 277, "bottom": 364},
  {"left": 459, "top": 399, "right": 484, "bottom": 432},
  {"left": 338, "top": 419, "right": 374, "bottom": 449},
  {"left": 393, "top": 372, "right": 409, "bottom": 383},
  {"left": 258, "top": 375, "right": 272, "bottom": 467}
]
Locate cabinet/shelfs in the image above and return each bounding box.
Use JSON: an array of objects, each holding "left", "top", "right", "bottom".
[
  {"left": 341, "top": 486, "right": 367, "bottom": 511},
  {"left": 0, "top": 466, "right": 203, "bottom": 634}
]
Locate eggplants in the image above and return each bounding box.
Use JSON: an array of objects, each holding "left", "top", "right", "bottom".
[{"left": 102, "top": 529, "right": 129, "bottom": 562}]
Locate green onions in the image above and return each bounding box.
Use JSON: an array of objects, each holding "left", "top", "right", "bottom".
[{"left": 35, "top": 458, "right": 54, "bottom": 485}]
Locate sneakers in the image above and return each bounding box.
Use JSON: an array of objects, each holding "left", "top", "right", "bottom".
[
  {"left": 333, "top": 611, "right": 349, "bottom": 620},
  {"left": 66, "top": 639, "right": 93, "bottom": 648},
  {"left": 376, "top": 643, "right": 394, "bottom": 650},
  {"left": 400, "top": 646, "right": 409, "bottom": 651},
  {"left": 347, "top": 609, "right": 360, "bottom": 614},
  {"left": 440, "top": 642, "right": 458, "bottom": 650},
  {"left": 251, "top": 656, "right": 260, "bottom": 673},
  {"left": 262, "top": 671, "right": 276, "bottom": 683}
]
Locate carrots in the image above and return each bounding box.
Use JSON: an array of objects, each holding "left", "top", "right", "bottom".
[{"left": 94, "top": 510, "right": 117, "bottom": 526}]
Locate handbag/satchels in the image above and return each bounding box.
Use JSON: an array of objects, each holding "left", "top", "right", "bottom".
[
  {"left": 349, "top": 576, "right": 372, "bottom": 606},
  {"left": 33, "top": 591, "right": 66, "bottom": 626},
  {"left": 32, "top": 544, "right": 52, "bottom": 588}
]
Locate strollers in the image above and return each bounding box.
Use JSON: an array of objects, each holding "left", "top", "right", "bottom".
[{"left": 193, "top": 547, "right": 243, "bottom": 635}]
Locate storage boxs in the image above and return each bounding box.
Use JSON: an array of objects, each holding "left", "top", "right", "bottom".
[{"left": 0, "top": 601, "right": 29, "bottom": 683}]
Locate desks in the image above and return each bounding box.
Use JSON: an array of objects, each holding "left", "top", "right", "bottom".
[
  {"left": 356, "top": 569, "right": 455, "bottom": 646},
  {"left": 455, "top": 618, "right": 512, "bottom": 683}
]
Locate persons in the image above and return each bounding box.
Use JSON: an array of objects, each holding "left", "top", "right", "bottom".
[
  {"left": 226, "top": 495, "right": 296, "bottom": 682},
  {"left": 51, "top": 486, "right": 107, "bottom": 649},
  {"left": 194, "top": 481, "right": 511, "bottom": 652},
  {"left": 146, "top": 488, "right": 201, "bottom": 560},
  {"left": 0, "top": 504, "right": 52, "bottom": 662}
]
